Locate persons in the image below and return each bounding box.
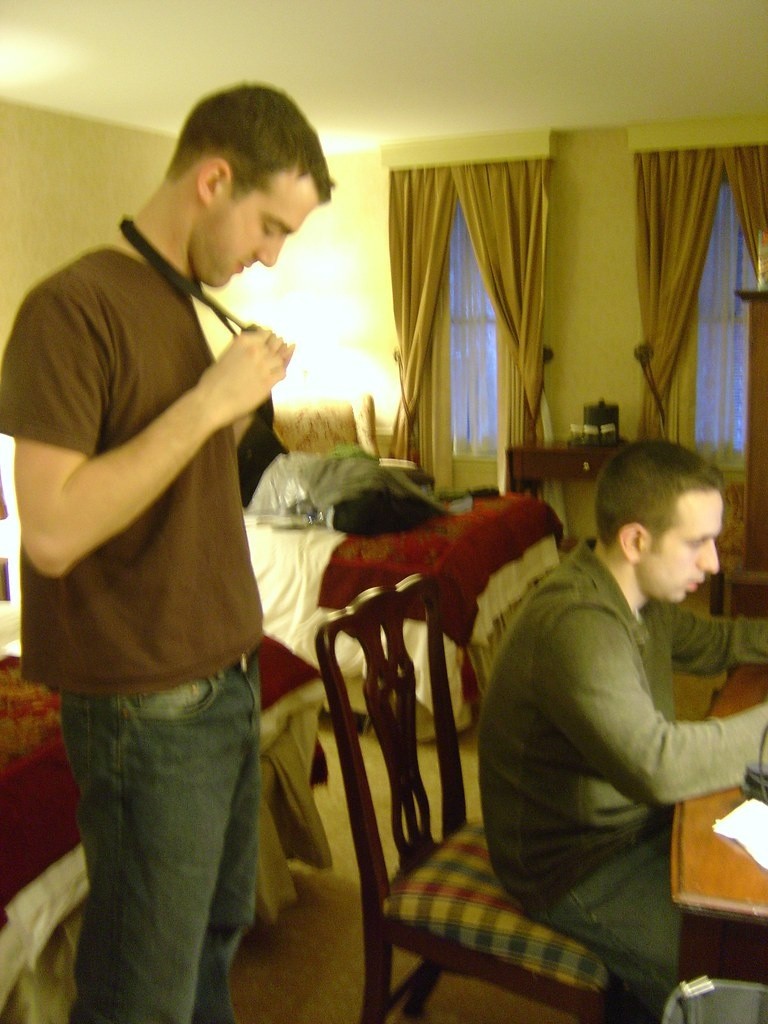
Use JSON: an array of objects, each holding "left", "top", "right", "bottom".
[
  {"left": 479, "top": 437, "right": 768, "bottom": 1024},
  {"left": 0, "top": 84, "right": 334, "bottom": 1024}
]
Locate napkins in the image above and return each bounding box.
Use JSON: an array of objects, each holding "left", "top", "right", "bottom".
[{"left": 712, "top": 797, "right": 768, "bottom": 872}]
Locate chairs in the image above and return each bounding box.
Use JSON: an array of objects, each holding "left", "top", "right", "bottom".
[{"left": 313, "top": 573, "right": 624, "bottom": 1024}]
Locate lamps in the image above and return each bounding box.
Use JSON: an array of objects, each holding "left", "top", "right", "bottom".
[{"left": 633, "top": 342, "right": 668, "bottom": 444}]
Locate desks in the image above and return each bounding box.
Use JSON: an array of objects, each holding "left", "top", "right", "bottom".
[
  {"left": 668, "top": 660, "right": 768, "bottom": 994},
  {"left": 507, "top": 441, "right": 633, "bottom": 501}
]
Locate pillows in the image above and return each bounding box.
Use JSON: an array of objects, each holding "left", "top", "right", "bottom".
[{"left": 297, "top": 448, "right": 449, "bottom": 535}]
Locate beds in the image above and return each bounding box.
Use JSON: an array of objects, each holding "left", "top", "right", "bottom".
[
  {"left": 0, "top": 523, "right": 329, "bottom": 1024},
  {"left": 227, "top": 393, "right": 566, "bottom": 747}
]
